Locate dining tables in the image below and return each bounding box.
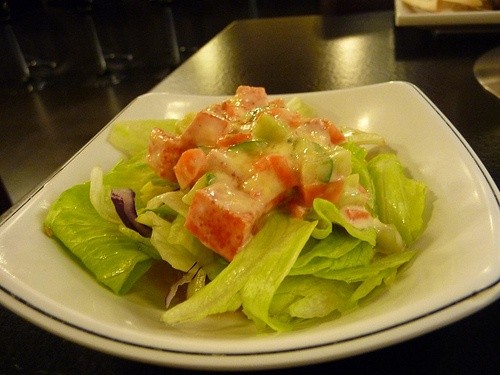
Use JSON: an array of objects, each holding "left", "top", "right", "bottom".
[{"left": 0, "top": 9, "right": 500, "bottom": 374}]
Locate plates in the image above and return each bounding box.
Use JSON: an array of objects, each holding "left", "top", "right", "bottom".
[
  {"left": 0, "top": 79, "right": 500, "bottom": 373},
  {"left": 394, "top": 0, "right": 499, "bottom": 31}
]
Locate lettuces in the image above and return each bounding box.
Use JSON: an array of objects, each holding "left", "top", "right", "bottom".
[{"left": 40, "top": 95, "right": 433, "bottom": 337}]
